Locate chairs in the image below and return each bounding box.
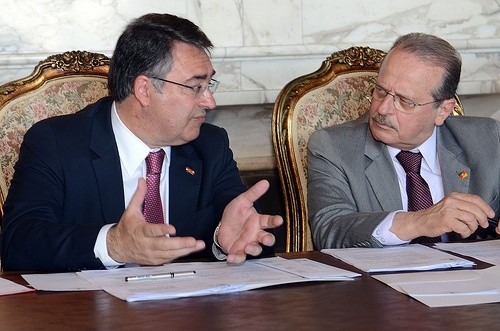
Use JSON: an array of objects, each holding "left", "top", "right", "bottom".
[
  {"left": 271, "top": 46, "right": 466, "bottom": 253},
  {"left": 0, "top": 51, "right": 112, "bottom": 217}
]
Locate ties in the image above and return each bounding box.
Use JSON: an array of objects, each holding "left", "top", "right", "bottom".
[
  {"left": 144, "top": 149, "right": 168, "bottom": 267},
  {"left": 396, "top": 150, "right": 443, "bottom": 244}
]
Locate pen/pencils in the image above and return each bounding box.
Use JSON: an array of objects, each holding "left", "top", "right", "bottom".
[
  {"left": 487, "top": 218, "right": 498, "bottom": 226},
  {"left": 125, "top": 270, "right": 196, "bottom": 282}
]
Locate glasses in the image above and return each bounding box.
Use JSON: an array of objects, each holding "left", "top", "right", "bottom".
[
  {"left": 373, "top": 82, "right": 447, "bottom": 112},
  {"left": 146, "top": 77, "right": 220, "bottom": 96}
]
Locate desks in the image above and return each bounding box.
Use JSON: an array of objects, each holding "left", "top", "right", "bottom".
[{"left": 0, "top": 249, "right": 500, "bottom": 331}]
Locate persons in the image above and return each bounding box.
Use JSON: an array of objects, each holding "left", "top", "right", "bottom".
[
  {"left": 0, "top": 12, "right": 284, "bottom": 272},
  {"left": 306, "top": 33, "right": 500, "bottom": 250}
]
